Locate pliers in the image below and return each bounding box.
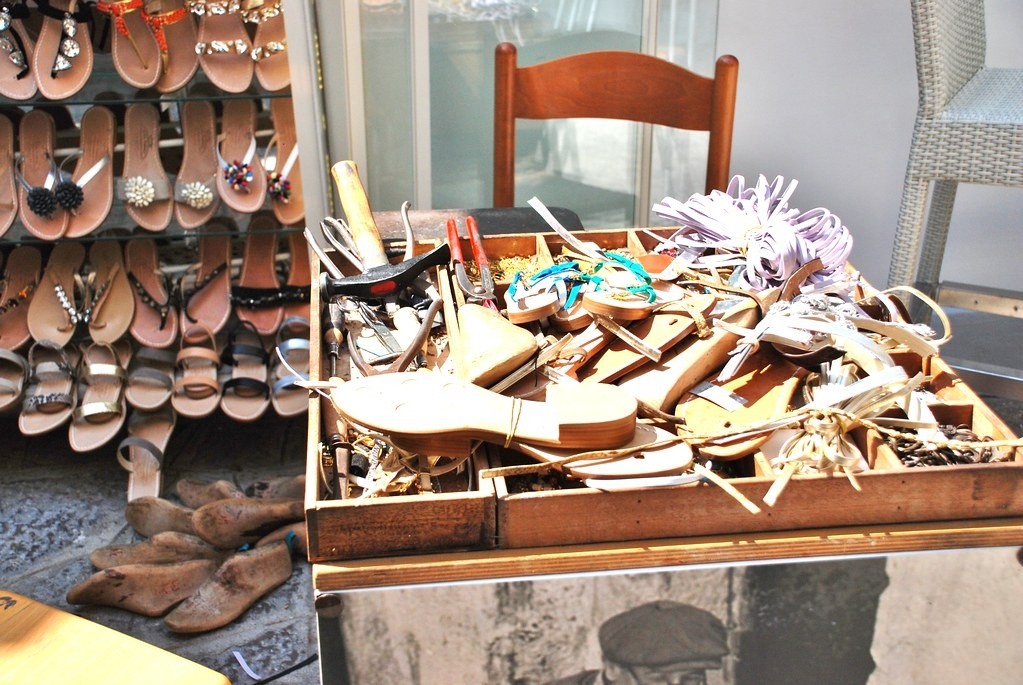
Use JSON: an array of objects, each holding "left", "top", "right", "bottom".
[
  {"left": 447, "top": 216, "right": 496, "bottom": 299},
  {"left": 320, "top": 200, "right": 440, "bottom": 318}
]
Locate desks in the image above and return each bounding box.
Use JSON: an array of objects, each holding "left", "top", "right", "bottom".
[{"left": 309, "top": 222, "right": 1023, "bottom": 685}]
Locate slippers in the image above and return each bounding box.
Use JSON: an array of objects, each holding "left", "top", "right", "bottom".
[{"left": 0, "top": 0, "right": 311, "bottom": 501}]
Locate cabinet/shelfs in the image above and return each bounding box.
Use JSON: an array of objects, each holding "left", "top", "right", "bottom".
[{"left": 0, "top": 0, "right": 310, "bottom": 453}]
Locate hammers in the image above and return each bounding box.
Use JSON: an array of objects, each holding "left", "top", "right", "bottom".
[{"left": 318, "top": 161, "right": 452, "bottom": 303}]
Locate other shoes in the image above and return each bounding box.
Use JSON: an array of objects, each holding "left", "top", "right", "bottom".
[{"left": 320, "top": 246, "right": 966, "bottom": 488}]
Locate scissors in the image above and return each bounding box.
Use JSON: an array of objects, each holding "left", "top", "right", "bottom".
[{"left": 336, "top": 295, "right": 402, "bottom": 354}]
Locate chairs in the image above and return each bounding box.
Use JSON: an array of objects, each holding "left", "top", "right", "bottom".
[
  {"left": 886, "top": 0, "right": 1023, "bottom": 402},
  {"left": 491, "top": 41, "right": 739, "bottom": 211}
]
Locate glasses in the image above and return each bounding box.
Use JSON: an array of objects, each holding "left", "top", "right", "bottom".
[{"left": 628, "top": 667, "right": 707, "bottom": 685}]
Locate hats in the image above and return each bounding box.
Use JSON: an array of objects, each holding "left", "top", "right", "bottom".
[{"left": 598, "top": 599, "right": 730, "bottom": 672}]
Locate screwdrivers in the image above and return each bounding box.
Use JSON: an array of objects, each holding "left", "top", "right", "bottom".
[{"left": 322, "top": 301, "right": 345, "bottom": 378}]
